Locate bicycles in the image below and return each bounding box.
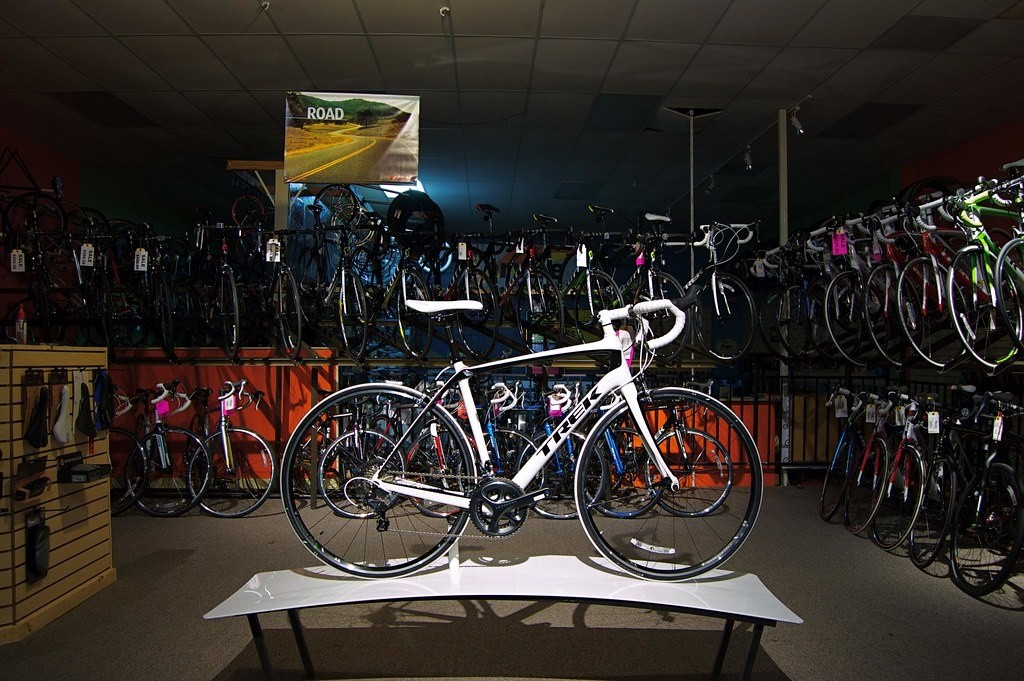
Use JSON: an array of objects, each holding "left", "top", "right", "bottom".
[
  {"left": 0, "top": 158, "right": 1024, "bottom": 602},
  {"left": 274, "top": 297, "right": 766, "bottom": 584}
]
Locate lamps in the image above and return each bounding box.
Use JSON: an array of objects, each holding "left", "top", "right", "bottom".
[
  {"left": 743, "top": 148, "right": 752, "bottom": 171},
  {"left": 667, "top": 206, "right": 673, "bottom": 221},
  {"left": 790, "top": 110, "right": 804, "bottom": 136},
  {"left": 705, "top": 174, "right": 715, "bottom": 196}
]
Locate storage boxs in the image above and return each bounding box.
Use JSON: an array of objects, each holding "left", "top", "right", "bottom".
[{"left": 56, "top": 462, "right": 111, "bottom": 482}]
